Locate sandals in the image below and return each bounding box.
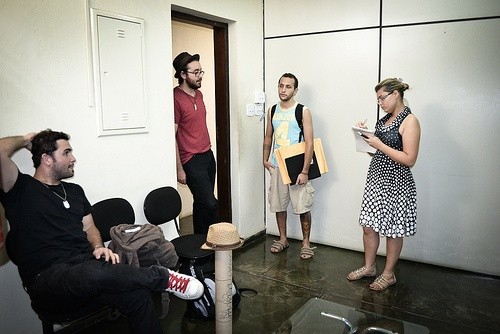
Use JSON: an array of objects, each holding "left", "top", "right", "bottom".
[
  {"left": 369, "top": 271, "right": 397, "bottom": 291},
  {"left": 346, "top": 263, "right": 377, "bottom": 281}
]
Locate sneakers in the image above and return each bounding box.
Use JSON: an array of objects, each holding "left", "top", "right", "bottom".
[{"left": 160, "top": 267, "right": 205, "bottom": 300}]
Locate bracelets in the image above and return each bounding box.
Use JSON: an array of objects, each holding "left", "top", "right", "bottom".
[{"left": 301, "top": 171, "right": 308, "bottom": 175}]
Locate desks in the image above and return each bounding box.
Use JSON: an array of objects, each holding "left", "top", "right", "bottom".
[{"left": 272, "top": 298, "right": 429, "bottom": 334}]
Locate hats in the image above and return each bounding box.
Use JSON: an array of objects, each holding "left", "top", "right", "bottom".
[
  {"left": 172, "top": 51, "right": 200, "bottom": 78},
  {"left": 200, "top": 222, "right": 245, "bottom": 250}
]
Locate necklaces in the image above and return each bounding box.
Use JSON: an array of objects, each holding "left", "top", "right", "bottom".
[
  {"left": 31, "top": 175, "right": 70, "bottom": 209},
  {"left": 179, "top": 85, "right": 197, "bottom": 111}
]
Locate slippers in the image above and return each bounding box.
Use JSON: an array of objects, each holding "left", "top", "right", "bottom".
[
  {"left": 300, "top": 246, "right": 315, "bottom": 260},
  {"left": 270, "top": 240, "right": 290, "bottom": 253}
]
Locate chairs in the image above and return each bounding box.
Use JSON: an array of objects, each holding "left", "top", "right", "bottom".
[
  {"left": 143, "top": 187, "right": 240, "bottom": 321},
  {"left": 91, "top": 198, "right": 135, "bottom": 242},
  {"left": 5, "top": 231, "right": 115, "bottom": 334}
]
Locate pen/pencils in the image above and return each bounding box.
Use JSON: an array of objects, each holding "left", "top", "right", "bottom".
[
  {"left": 358, "top": 131, "right": 369, "bottom": 139},
  {"left": 362, "top": 119, "right": 367, "bottom": 126}
]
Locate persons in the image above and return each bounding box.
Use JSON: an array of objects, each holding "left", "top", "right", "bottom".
[
  {"left": 0, "top": 130, "right": 205, "bottom": 334},
  {"left": 262, "top": 73, "right": 317, "bottom": 261},
  {"left": 347, "top": 77, "right": 421, "bottom": 291},
  {"left": 174, "top": 53, "right": 219, "bottom": 234}
]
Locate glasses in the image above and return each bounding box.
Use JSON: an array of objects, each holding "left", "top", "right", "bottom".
[
  {"left": 376, "top": 92, "right": 391, "bottom": 104},
  {"left": 186, "top": 70, "right": 204, "bottom": 77}
]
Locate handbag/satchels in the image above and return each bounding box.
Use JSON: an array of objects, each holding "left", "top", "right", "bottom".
[
  {"left": 186, "top": 273, "right": 242, "bottom": 323},
  {"left": 108, "top": 223, "right": 179, "bottom": 270}
]
